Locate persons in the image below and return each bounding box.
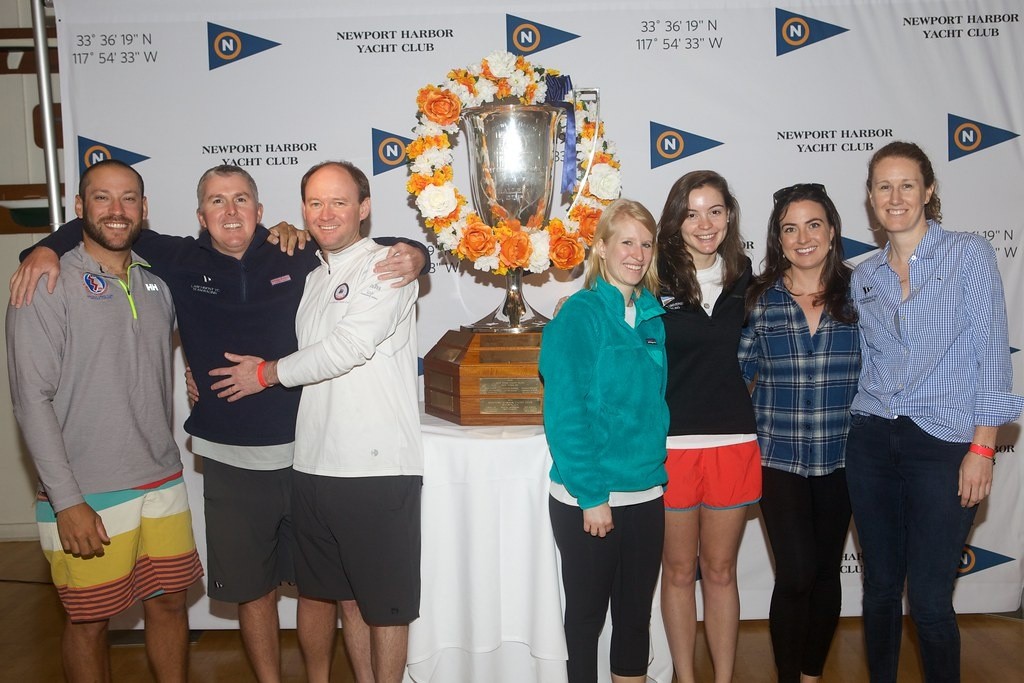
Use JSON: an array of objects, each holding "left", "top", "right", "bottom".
[
  {"left": 539, "top": 198, "right": 671, "bottom": 683},
  {"left": 737, "top": 182, "right": 861, "bottom": 683},
  {"left": 10, "top": 165, "right": 432, "bottom": 683},
  {"left": 183, "top": 160, "right": 424, "bottom": 683},
  {"left": 843, "top": 141, "right": 1024, "bottom": 683},
  {"left": 5, "top": 159, "right": 204, "bottom": 683},
  {"left": 554, "top": 170, "right": 763, "bottom": 683}
]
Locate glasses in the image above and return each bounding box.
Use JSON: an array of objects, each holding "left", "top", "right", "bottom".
[{"left": 772, "top": 183, "right": 828, "bottom": 208}]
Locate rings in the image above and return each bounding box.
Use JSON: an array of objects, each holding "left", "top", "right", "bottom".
[{"left": 230, "top": 387, "right": 234, "bottom": 394}]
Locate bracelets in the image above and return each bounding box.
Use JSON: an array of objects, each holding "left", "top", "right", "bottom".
[
  {"left": 257, "top": 361, "right": 270, "bottom": 387},
  {"left": 969, "top": 444, "right": 995, "bottom": 461}
]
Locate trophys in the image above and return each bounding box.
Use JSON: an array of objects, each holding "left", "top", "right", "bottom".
[{"left": 455, "top": 88, "right": 600, "bottom": 333}]
